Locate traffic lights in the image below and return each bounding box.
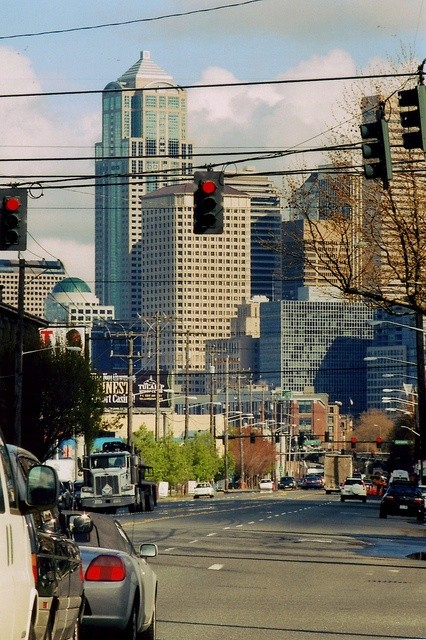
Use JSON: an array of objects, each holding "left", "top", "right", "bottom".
[
  {"left": 192, "top": 171, "right": 224, "bottom": 234},
  {"left": 398, "top": 85, "right": 426, "bottom": 153},
  {"left": 351, "top": 438, "right": 357, "bottom": 449},
  {"left": 0, "top": 188, "right": 26, "bottom": 251},
  {"left": 358, "top": 118, "right": 393, "bottom": 181},
  {"left": 376, "top": 437, "right": 382, "bottom": 448},
  {"left": 298, "top": 437, "right": 303, "bottom": 448}
]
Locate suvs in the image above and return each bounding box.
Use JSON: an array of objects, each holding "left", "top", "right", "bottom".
[{"left": 339, "top": 476, "right": 367, "bottom": 504}]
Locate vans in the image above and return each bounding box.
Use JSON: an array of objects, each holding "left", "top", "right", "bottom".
[{"left": 388, "top": 469, "right": 411, "bottom": 488}]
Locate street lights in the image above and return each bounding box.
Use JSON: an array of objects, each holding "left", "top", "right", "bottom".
[
  {"left": 385, "top": 407, "right": 414, "bottom": 419},
  {"left": 367, "top": 318, "right": 426, "bottom": 335},
  {"left": 362, "top": 356, "right": 426, "bottom": 366},
  {"left": 381, "top": 397, "right": 419, "bottom": 406},
  {"left": 381, "top": 373, "right": 419, "bottom": 381},
  {"left": 400, "top": 425, "right": 422, "bottom": 437},
  {"left": 154, "top": 395, "right": 198, "bottom": 453},
  {"left": 127, "top": 388, "right": 174, "bottom": 454},
  {"left": 382, "top": 389, "right": 419, "bottom": 398},
  {"left": 382, "top": 400, "right": 418, "bottom": 407},
  {"left": 222, "top": 410, "right": 298, "bottom": 491},
  {"left": 182, "top": 401, "right": 222, "bottom": 447},
  {"left": 14, "top": 345, "right": 83, "bottom": 445}
]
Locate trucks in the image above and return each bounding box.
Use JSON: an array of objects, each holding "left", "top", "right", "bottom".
[
  {"left": 75, "top": 441, "right": 159, "bottom": 512},
  {"left": 39, "top": 435, "right": 126, "bottom": 509},
  {"left": 322, "top": 453, "right": 353, "bottom": 494}
]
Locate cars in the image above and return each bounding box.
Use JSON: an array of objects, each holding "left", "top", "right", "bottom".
[
  {"left": 418, "top": 485, "right": 426, "bottom": 512},
  {"left": 379, "top": 479, "right": 425, "bottom": 521},
  {"left": 353, "top": 472, "right": 362, "bottom": 478},
  {"left": 0, "top": 436, "right": 60, "bottom": 640},
  {"left": 5, "top": 443, "right": 96, "bottom": 640},
  {"left": 278, "top": 476, "right": 298, "bottom": 491},
  {"left": 294, "top": 478, "right": 303, "bottom": 488},
  {"left": 302, "top": 473, "right": 323, "bottom": 490},
  {"left": 193, "top": 482, "right": 214, "bottom": 499},
  {"left": 258, "top": 478, "right": 275, "bottom": 491},
  {"left": 55, "top": 507, "right": 160, "bottom": 639}
]
